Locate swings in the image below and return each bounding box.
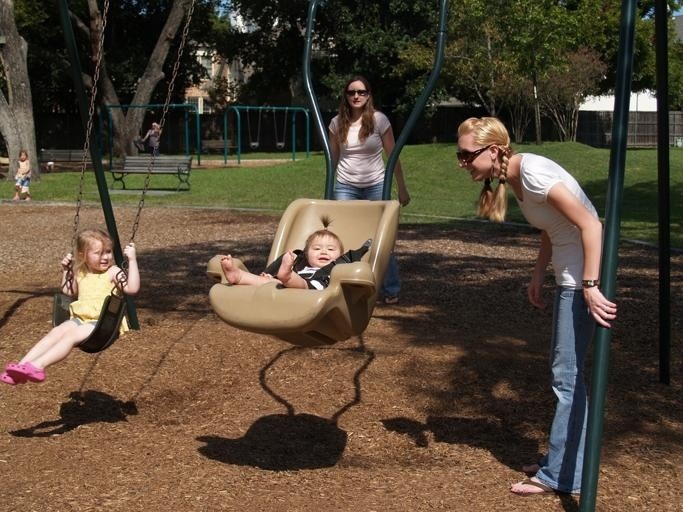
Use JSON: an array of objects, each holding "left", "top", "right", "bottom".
[
  {"left": 207, "top": 0, "right": 446, "bottom": 346},
  {"left": 53, "top": 0, "right": 195, "bottom": 353}
]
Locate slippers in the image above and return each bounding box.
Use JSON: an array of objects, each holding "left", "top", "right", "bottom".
[
  {"left": 0, "top": 372, "right": 27, "bottom": 385},
  {"left": 383, "top": 292, "right": 399, "bottom": 305},
  {"left": 516, "top": 477, "right": 558, "bottom": 497},
  {"left": 6, "top": 362, "right": 45, "bottom": 382}
]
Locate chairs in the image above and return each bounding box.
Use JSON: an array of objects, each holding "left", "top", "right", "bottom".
[{"left": 203, "top": 196, "right": 401, "bottom": 350}]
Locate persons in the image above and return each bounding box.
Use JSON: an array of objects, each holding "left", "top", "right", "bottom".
[
  {"left": 219, "top": 214, "right": 373, "bottom": 290},
  {"left": 14, "top": 150, "right": 32, "bottom": 202},
  {"left": 329, "top": 75, "right": 411, "bottom": 303},
  {"left": 142, "top": 122, "right": 160, "bottom": 156},
  {"left": 1, "top": 229, "right": 140, "bottom": 385},
  {"left": 456, "top": 116, "right": 618, "bottom": 495}
]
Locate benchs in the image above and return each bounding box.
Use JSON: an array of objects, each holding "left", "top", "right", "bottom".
[
  {"left": 199, "top": 139, "right": 239, "bottom": 153},
  {"left": 37, "top": 148, "right": 92, "bottom": 174},
  {"left": 107, "top": 155, "right": 193, "bottom": 193}
]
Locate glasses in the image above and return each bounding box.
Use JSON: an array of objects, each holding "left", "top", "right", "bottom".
[
  {"left": 455, "top": 145, "right": 490, "bottom": 163},
  {"left": 346, "top": 90, "right": 368, "bottom": 96}
]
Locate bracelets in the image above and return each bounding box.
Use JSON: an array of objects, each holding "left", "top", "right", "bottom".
[{"left": 582, "top": 279, "right": 600, "bottom": 287}]
